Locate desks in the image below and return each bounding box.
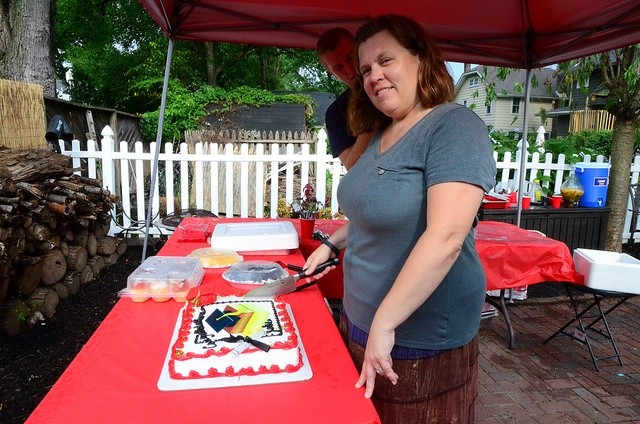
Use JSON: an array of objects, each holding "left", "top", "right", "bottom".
[
  {"left": 542, "top": 276, "right": 636, "bottom": 373},
  {"left": 158, "top": 216, "right": 358, "bottom": 300},
  {"left": 468, "top": 222, "right": 563, "bottom": 350},
  {"left": 22, "top": 255, "right": 381, "bottom": 423}
]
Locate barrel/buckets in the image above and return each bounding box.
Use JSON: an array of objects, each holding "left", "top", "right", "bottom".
[{"left": 574, "top": 162, "right": 613, "bottom": 209}]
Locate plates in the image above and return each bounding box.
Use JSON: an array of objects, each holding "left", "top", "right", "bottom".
[
  {"left": 187, "top": 248, "right": 244, "bottom": 268},
  {"left": 222, "top": 260, "right": 289, "bottom": 290}
]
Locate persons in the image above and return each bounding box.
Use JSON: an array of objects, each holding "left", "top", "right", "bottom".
[
  {"left": 314, "top": 25, "right": 382, "bottom": 171},
  {"left": 301, "top": 10, "right": 501, "bottom": 424}
]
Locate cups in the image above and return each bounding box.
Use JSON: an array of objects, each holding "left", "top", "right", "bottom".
[
  {"left": 522, "top": 196, "right": 530, "bottom": 210},
  {"left": 509, "top": 192, "right": 518, "bottom": 208},
  {"left": 552, "top": 195, "right": 562, "bottom": 209},
  {"left": 300, "top": 219, "right": 315, "bottom": 238}
]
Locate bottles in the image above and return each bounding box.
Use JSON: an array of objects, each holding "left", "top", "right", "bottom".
[{"left": 530, "top": 179, "right": 543, "bottom": 205}]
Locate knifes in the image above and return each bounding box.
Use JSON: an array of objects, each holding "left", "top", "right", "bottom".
[{"left": 243, "top": 259, "right": 341, "bottom": 299}]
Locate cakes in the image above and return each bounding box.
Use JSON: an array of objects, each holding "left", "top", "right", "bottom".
[{"left": 157, "top": 281, "right": 315, "bottom": 391}]
